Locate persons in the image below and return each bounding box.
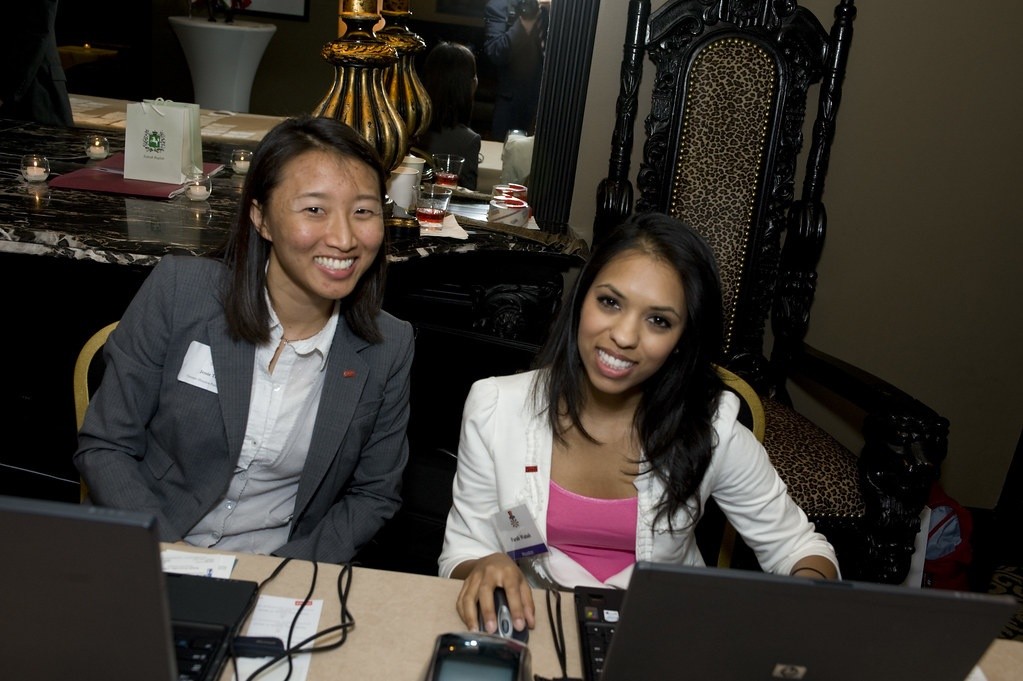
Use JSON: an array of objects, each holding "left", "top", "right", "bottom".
[
  {"left": 499, "top": 123, "right": 537, "bottom": 192},
  {"left": 74, "top": 116, "right": 418, "bottom": 567},
  {"left": 482, "top": 0, "right": 552, "bottom": 116},
  {"left": 435, "top": 215, "right": 843, "bottom": 637},
  {"left": 0, "top": 0, "right": 77, "bottom": 130},
  {"left": 417, "top": 40, "right": 481, "bottom": 191}
]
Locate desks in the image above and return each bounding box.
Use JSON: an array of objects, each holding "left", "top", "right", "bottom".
[
  {"left": 159, "top": 541, "right": 1023, "bottom": 681},
  {"left": 168, "top": 15, "right": 277, "bottom": 113},
  {"left": 68, "top": 93, "right": 503, "bottom": 195}
]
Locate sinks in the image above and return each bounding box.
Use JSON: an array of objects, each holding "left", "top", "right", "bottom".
[{"left": 170, "top": 17, "right": 277, "bottom": 110}]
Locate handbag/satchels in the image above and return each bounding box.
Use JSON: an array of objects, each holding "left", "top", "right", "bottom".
[{"left": 124, "top": 97, "right": 204, "bottom": 185}]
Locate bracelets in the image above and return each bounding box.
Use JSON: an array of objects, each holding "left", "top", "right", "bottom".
[{"left": 791, "top": 567, "right": 828, "bottom": 581}]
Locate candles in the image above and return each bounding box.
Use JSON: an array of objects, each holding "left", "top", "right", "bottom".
[
  {"left": 183, "top": 172, "right": 212, "bottom": 202},
  {"left": 20, "top": 154, "right": 50, "bottom": 183},
  {"left": 230, "top": 149, "right": 253, "bottom": 176},
  {"left": 84, "top": 135, "right": 110, "bottom": 160}
]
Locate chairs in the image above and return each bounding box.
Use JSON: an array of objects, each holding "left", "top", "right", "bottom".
[
  {"left": 73, "top": 321, "right": 119, "bottom": 503},
  {"left": 590, "top": 0, "right": 951, "bottom": 586}
]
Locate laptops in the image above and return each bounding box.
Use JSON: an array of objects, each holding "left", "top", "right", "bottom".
[
  {"left": 0, "top": 494, "right": 260, "bottom": 681},
  {"left": 574, "top": 559, "right": 1015, "bottom": 681}
]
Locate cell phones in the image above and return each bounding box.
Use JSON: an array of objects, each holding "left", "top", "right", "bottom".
[{"left": 424, "top": 630, "right": 534, "bottom": 681}]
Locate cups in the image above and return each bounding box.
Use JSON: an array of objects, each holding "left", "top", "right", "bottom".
[
  {"left": 83, "top": 134, "right": 110, "bottom": 159},
  {"left": 414, "top": 185, "right": 452, "bottom": 226},
  {"left": 183, "top": 173, "right": 212, "bottom": 201},
  {"left": 230, "top": 149, "right": 255, "bottom": 174},
  {"left": 385, "top": 166, "right": 417, "bottom": 211},
  {"left": 433, "top": 153, "right": 466, "bottom": 185},
  {"left": 401, "top": 156, "right": 427, "bottom": 185},
  {"left": 20, "top": 153, "right": 51, "bottom": 182}
]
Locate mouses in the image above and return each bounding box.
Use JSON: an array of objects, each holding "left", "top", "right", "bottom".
[{"left": 475, "top": 585, "right": 529, "bottom": 645}]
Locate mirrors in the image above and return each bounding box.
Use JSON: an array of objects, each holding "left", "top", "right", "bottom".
[{"left": 0, "top": 0, "right": 601, "bottom": 235}]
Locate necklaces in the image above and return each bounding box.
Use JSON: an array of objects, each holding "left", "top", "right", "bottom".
[{"left": 282, "top": 324, "right": 323, "bottom": 346}]
